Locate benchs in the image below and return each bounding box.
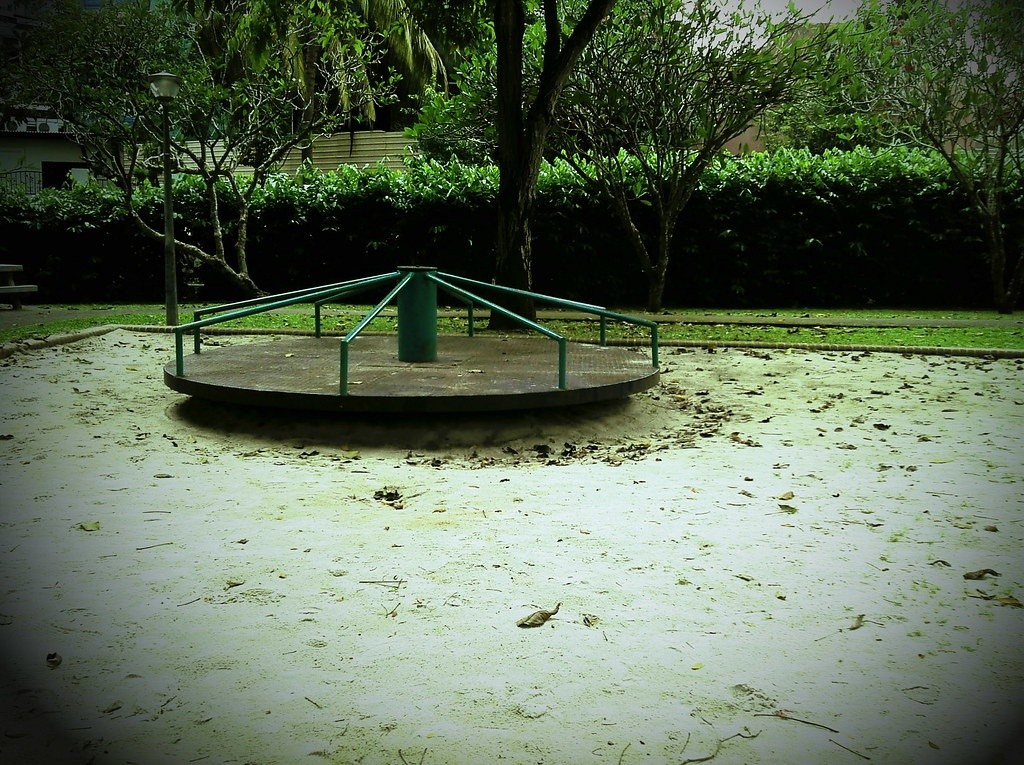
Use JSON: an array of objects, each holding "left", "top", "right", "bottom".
[{"left": 0, "top": 264, "right": 38, "bottom": 310}]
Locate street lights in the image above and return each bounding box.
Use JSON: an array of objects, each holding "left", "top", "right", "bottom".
[{"left": 149, "top": 67, "right": 190, "bottom": 327}]
